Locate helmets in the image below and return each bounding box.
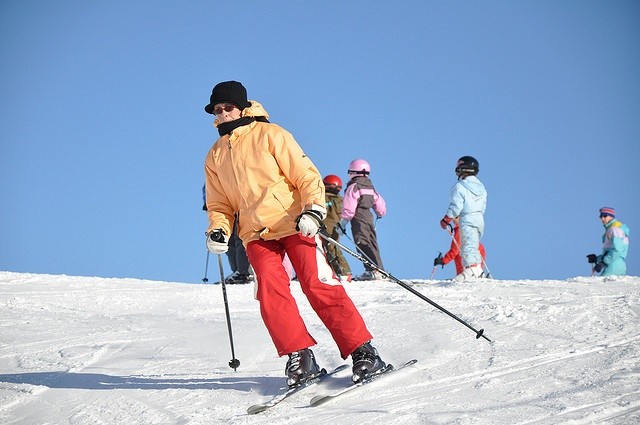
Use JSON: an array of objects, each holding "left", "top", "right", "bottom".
[
  {"left": 455, "top": 156, "right": 479, "bottom": 178},
  {"left": 323, "top": 175, "right": 342, "bottom": 194},
  {"left": 347, "top": 159, "right": 371, "bottom": 175}
]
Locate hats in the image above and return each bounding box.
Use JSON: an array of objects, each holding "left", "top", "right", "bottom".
[
  {"left": 205, "top": 81, "right": 247, "bottom": 113},
  {"left": 599, "top": 207, "right": 615, "bottom": 217}
]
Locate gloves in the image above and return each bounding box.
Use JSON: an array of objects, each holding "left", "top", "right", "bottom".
[
  {"left": 587, "top": 254, "right": 597, "bottom": 263},
  {"left": 594, "top": 261, "right": 604, "bottom": 272},
  {"left": 433, "top": 257, "right": 444, "bottom": 268},
  {"left": 298, "top": 203, "right": 328, "bottom": 238},
  {"left": 440, "top": 216, "right": 452, "bottom": 230},
  {"left": 335, "top": 217, "right": 349, "bottom": 235},
  {"left": 206, "top": 229, "right": 230, "bottom": 254}
]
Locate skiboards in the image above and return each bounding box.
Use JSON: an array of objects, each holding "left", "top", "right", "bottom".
[{"left": 247, "top": 359, "right": 418, "bottom": 414}]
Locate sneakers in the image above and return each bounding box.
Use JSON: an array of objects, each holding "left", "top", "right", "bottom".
[
  {"left": 285, "top": 352, "right": 313, "bottom": 377},
  {"left": 225, "top": 271, "right": 248, "bottom": 284},
  {"left": 453, "top": 267, "right": 477, "bottom": 279},
  {"left": 357, "top": 270, "right": 382, "bottom": 280},
  {"left": 352, "top": 347, "right": 377, "bottom": 373}
]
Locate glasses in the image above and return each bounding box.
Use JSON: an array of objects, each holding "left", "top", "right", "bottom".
[
  {"left": 211, "top": 106, "right": 234, "bottom": 114},
  {"left": 600, "top": 215, "right": 607, "bottom": 218}
]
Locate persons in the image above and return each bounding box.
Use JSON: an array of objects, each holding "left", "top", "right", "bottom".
[
  {"left": 226, "top": 222, "right": 251, "bottom": 282},
  {"left": 334, "top": 158, "right": 387, "bottom": 279},
  {"left": 441, "top": 156, "right": 488, "bottom": 278},
  {"left": 320, "top": 175, "right": 352, "bottom": 277},
  {"left": 434, "top": 216, "right": 486, "bottom": 276},
  {"left": 204, "top": 80, "right": 378, "bottom": 377},
  {"left": 586, "top": 206, "right": 630, "bottom": 276}
]
